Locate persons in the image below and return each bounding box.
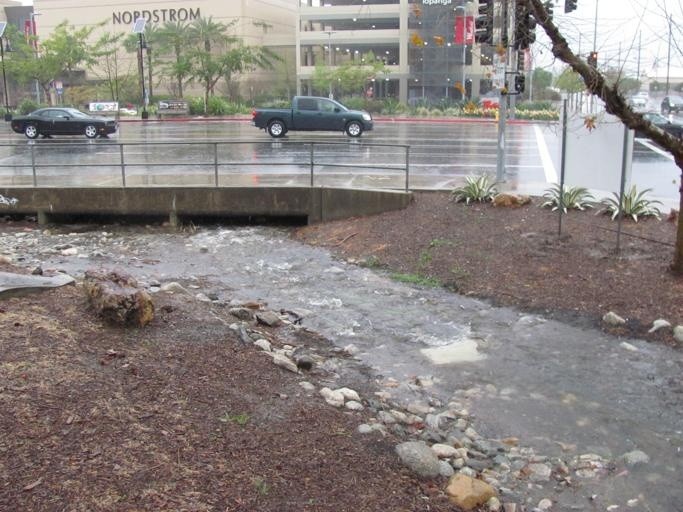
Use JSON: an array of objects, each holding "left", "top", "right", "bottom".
[{"left": 367, "top": 86, "right": 374, "bottom": 98}]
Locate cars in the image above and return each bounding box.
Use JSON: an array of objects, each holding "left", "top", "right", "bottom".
[
  {"left": 12, "top": 107, "right": 116, "bottom": 138},
  {"left": 661, "top": 95, "right": 682, "bottom": 114},
  {"left": 629, "top": 91, "right": 649, "bottom": 106}
]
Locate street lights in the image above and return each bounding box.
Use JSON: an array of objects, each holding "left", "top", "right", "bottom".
[
  {"left": 456, "top": 4, "right": 467, "bottom": 102},
  {"left": 30, "top": 11, "right": 43, "bottom": 104}
]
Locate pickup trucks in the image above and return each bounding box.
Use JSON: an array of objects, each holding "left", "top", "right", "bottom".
[{"left": 253, "top": 96, "right": 373, "bottom": 137}]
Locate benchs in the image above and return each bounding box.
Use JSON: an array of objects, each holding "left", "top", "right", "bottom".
[
  {"left": 86, "top": 101, "right": 121, "bottom": 121},
  {"left": 156, "top": 99, "right": 191, "bottom": 120}
]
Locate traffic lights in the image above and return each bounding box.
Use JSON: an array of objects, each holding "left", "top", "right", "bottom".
[
  {"left": 474, "top": 0, "right": 493, "bottom": 42},
  {"left": 545, "top": 1, "right": 553, "bottom": 21},
  {"left": 515, "top": 0, "right": 535, "bottom": 50},
  {"left": 565, "top": 1, "right": 576, "bottom": 13},
  {"left": 515, "top": 75, "right": 524, "bottom": 93},
  {"left": 587, "top": 53, "right": 597, "bottom": 67}
]
